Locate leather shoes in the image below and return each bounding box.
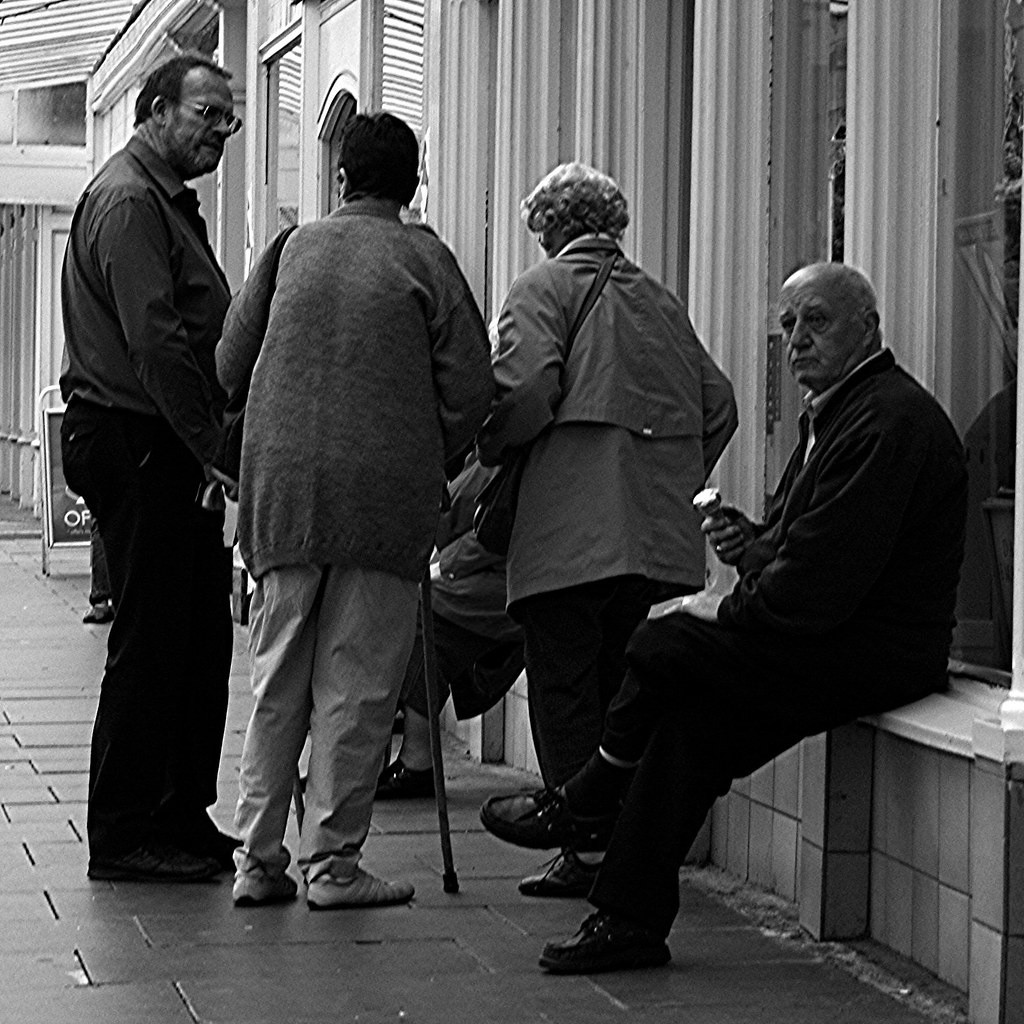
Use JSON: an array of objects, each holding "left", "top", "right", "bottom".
[
  {"left": 179, "top": 832, "right": 244, "bottom": 871},
  {"left": 370, "top": 759, "right": 435, "bottom": 800}
]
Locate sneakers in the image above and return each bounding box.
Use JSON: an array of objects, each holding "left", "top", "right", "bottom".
[
  {"left": 517, "top": 849, "right": 602, "bottom": 897},
  {"left": 86, "top": 838, "right": 220, "bottom": 879},
  {"left": 231, "top": 871, "right": 298, "bottom": 906},
  {"left": 538, "top": 908, "right": 673, "bottom": 973},
  {"left": 479, "top": 786, "right": 621, "bottom": 853},
  {"left": 305, "top": 867, "right": 414, "bottom": 911}
]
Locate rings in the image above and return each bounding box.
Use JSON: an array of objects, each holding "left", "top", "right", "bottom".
[{"left": 716, "top": 545, "right": 722, "bottom": 553}]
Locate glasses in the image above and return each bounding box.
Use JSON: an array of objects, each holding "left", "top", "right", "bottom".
[{"left": 171, "top": 97, "right": 245, "bottom": 133}]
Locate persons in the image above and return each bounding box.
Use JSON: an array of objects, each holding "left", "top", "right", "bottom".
[
  {"left": 378, "top": 315, "right": 525, "bottom": 802},
  {"left": 60, "top": 56, "right": 243, "bottom": 881},
  {"left": 953, "top": 377, "right": 1017, "bottom": 671},
  {"left": 478, "top": 162, "right": 740, "bottom": 895},
  {"left": 480, "top": 261, "right": 961, "bottom": 974},
  {"left": 84, "top": 519, "right": 114, "bottom": 625},
  {"left": 216, "top": 112, "right": 492, "bottom": 910}
]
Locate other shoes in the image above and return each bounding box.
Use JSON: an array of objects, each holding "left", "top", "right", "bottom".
[{"left": 82, "top": 604, "right": 114, "bottom": 623}]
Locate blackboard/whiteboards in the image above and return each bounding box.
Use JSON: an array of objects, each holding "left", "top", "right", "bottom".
[{"left": 42, "top": 408, "right": 95, "bottom": 549}]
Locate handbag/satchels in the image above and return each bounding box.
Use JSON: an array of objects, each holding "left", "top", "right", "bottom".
[
  {"left": 208, "top": 393, "right": 246, "bottom": 490},
  {"left": 471, "top": 457, "right": 524, "bottom": 555}
]
